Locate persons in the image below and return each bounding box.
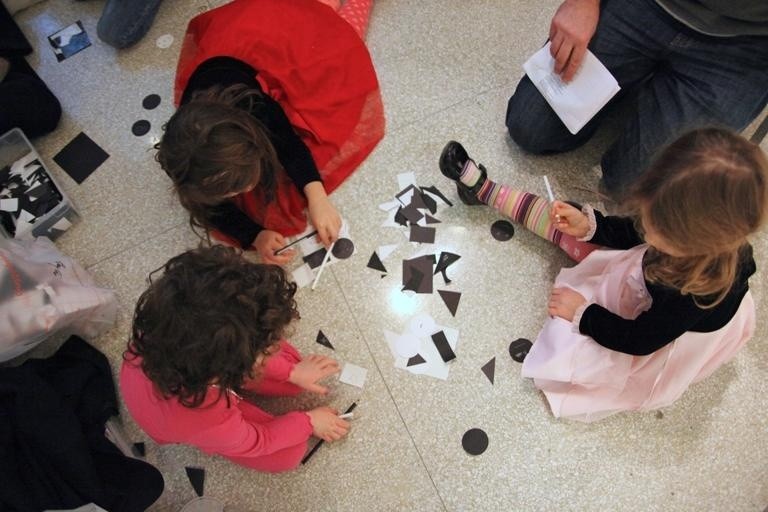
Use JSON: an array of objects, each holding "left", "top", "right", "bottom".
[
  {"left": 119, "top": 238, "right": 352, "bottom": 472},
  {"left": 504, "top": 1, "right": 767, "bottom": 203},
  {"left": 153, "top": 1, "right": 386, "bottom": 264},
  {"left": 438, "top": 124, "right": 768, "bottom": 425},
  {"left": 0, "top": 1, "right": 62, "bottom": 143},
  {"left": 95, "top": 0, "right": 160, "bottom": 49}
]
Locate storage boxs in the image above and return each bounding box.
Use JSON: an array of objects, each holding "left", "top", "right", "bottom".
[{"left": 0, "top": 127, "right": 81, "bottom": 251}]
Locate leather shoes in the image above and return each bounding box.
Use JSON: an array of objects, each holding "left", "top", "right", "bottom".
[{"left": 438, "top": 140, "right": 491, "bottom": 207}]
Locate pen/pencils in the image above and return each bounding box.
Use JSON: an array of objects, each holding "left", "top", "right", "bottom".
[
  {"left": 302, "top": 399, "right": 360, "bottom": 464},
  {"left": 311, "top": 242, "right": 335, "bottom": 290},
  {"left": 274, "top": 230, "right": 318, "bottom": 255},
  {"left": 543, "top": 175, "right": 561, "bottom": 223}
]
